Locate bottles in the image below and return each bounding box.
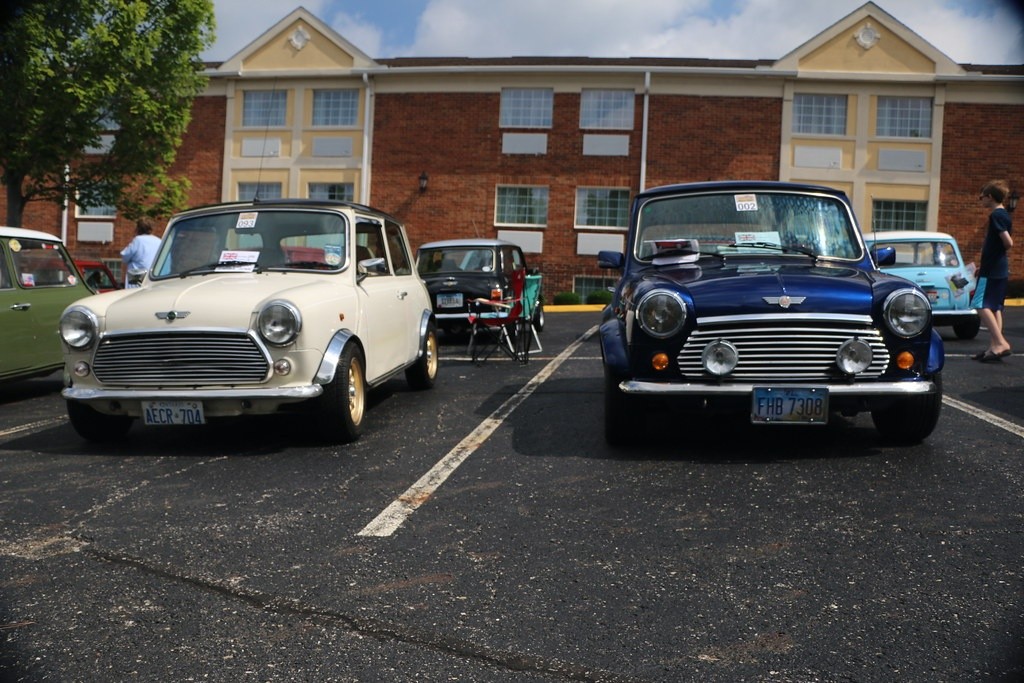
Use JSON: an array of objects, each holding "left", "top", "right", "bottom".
[{"left": 492, "top": 277, "right": 515, "bottom": 313}]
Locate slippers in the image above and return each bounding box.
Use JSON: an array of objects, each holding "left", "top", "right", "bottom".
[{"left": 970, "top": 349, "right": 1012, "bottom": 363}]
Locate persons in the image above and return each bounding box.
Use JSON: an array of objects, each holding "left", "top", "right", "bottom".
[
  {"left": 119, "top": 218, "right": 171, "bottom": 289},
  {"left": 970, "top": 181, "right": 1013, "bottom": 362}
]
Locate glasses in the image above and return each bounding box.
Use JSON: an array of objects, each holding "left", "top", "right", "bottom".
[{"left": 979, "top": 195, "right": 989, "bottom": 200}]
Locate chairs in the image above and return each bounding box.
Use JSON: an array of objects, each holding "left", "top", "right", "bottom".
[
  {"left": 357, "top": 244, "right": 376, "bottom": 260},
  {"left": 466, "top": 266, "right": 542, "bottom": 366},
  {"left": 434, "top": 252, "right": 459, "bottom": 271}
]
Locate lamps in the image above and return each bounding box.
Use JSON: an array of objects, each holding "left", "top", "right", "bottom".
[
  {"left": 419, "top": 170, "right": 428, "bottom": 191},
  {"left": 1007, "top": 190, "right": 1020, "bottom": 212}
]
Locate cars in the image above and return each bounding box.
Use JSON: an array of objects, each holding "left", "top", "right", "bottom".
[
  {"left": 861, "top": 231, "right": 981, "bottom": 337},
  {"left": 0, "top": 226, "right": 97, "bottom": 380},
  {"left": 598, "top": 179, "right": 945, "bottom": 449},
  {"left": 58, "top": 193, "right": 439, "bottom": 444},
  {"left": 413, "top": 238, "right": 544, "bottom": 341},
  {"left": 23, "top": 256, "right": 120, "bottom": 293}
]
